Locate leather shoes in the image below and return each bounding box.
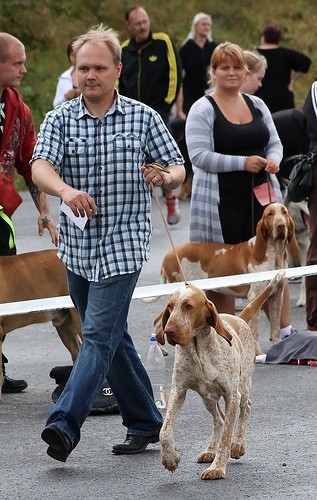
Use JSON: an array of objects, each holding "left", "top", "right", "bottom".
[
  {"left": 112, "top": 434, "right": 162, "bottom": 453},
  {"left": 41, "top": 423, "right": 72, "bottom": 462}
]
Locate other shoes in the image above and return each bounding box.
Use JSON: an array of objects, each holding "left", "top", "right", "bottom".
[
  {"left": 288, "top": 277, "right": 302, "bottom": 283},
  {"left": 281, "top": 328, "right": 298, "bottom": 340}
]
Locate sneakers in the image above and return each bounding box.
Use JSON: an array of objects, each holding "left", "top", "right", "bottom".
[
  {"left": 1, "top": 374, "right": 28, "bottom": 393},
  {"left": 165, "top": 195, "right": 180, "bottom": 225}
]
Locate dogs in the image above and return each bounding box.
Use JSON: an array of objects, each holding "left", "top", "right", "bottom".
[
  {"left": 140, "top": 203, "right": 300, "bottom": 363},
  {"left": 0, "top": 250, "right": 82, "bottom": 405},
  {"left": 153, "top": 284, "right": 255, "bottom": 481}
]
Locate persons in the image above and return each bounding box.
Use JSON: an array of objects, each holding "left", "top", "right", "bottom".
[
  {"left": 51, "top": 37, "right": 82, "bottom": 108},
  {"left": 203, "top": 50, "right": 267, "bottom": 98},
  {"left": 30, "top": 23, "right": 186, "bottom": 462},
  {"left": 176, "top": 13, "right": 219, "bottom": 201},
  {"left": 115, "top": 6, "right": 184, "bottom": 225},
  {"left": 255, "top": 25, "right": 312, "bottom": 191},
  {"left": 303, "top": 78, "right": 317, "bottom": 331},
  {"left": 0, "top": 30, "right": 60, "bottom": 394},
  {"left": 185, "top": 40, "right": 297, "bottom": 362}
]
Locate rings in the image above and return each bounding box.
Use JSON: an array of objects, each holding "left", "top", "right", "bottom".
[{"left": 154, "top": 177, "right": 159, "bottom": 182}]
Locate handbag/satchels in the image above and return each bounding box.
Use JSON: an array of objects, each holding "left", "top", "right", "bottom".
[
  {"left": 49, "top": 365, "right": 120, "bottom": 415},
  {"left": 283, "top": 152, "right": 316, "bottom": 203}
]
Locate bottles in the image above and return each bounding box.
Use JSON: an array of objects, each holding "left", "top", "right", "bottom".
[{"left": 145, "top": 337, "right": 167, "bottom": 410}]
